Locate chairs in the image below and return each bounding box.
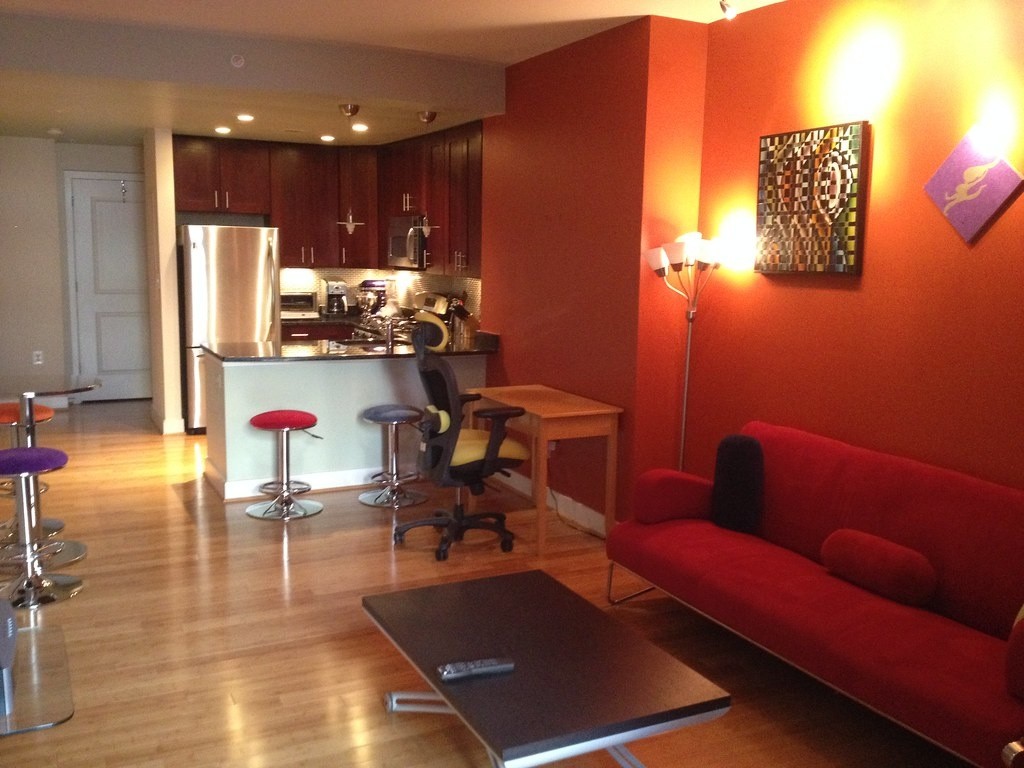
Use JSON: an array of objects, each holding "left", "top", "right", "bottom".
[{"left": 392, "top": 308, "right": 513, "bottom": 561}]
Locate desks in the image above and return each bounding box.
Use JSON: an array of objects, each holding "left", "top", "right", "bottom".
[
  {"left": 464, "top": 385, "right": 623, "bottom": 561},
  {"left": 0, "top": 377, "right": 102, "bottom": 574}
]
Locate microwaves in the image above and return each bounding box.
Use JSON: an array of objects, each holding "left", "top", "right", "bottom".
[{"left": 386, "top": 215, "right": 425, "bottom": 270}]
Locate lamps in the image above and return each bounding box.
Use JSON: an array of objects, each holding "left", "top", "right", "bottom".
[{"left": 650, "top": 230, "right": 732, "bottom": 470}]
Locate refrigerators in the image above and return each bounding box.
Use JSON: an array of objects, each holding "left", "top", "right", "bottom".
[{"left": 179, "top": 224, "right": 282, "bottom": 436}]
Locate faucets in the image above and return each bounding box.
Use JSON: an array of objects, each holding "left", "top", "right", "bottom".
[{"left": 386, "top": 320, "right": 392, "bottom": 348}]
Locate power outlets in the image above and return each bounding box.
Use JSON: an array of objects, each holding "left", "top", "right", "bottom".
[{"left": 34, "top": 350, "right": 43, "bottom": 365}]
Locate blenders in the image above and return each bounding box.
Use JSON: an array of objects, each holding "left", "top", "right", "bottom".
[{"left": 357, "top": 279, "right": 386, "bottom": 321}]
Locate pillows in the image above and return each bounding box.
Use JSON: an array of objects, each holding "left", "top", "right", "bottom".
[
  {"left": 816, "top": 529, "right": 935, "bottom": 601},
  {"left": 709, "top": 433, "right": 763, "bottom": 532},
  {"left": 1003, "top": 606, "right": 1024, "bottom": 698}
]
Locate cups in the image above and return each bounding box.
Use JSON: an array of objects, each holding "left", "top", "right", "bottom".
[{"left": 317, "top": 339, "right": 329, "bottom": 353}]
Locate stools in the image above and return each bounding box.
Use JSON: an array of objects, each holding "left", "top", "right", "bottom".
[
  {"left": 242, "top": 411, "right": 323, "bottom": 521},
  {"left": 355, "top": 402, "right": 422, "bottom": 511},
  {"left": 0, "top": 399, "right": 56, "bottom": 499},
  {"left": 0, "top": 448, "right": 88, "bottom": 607}
]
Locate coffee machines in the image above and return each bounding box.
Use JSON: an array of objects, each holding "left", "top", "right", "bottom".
[{"left": 320, "top": 276, "right": 349, "bottom": 318}]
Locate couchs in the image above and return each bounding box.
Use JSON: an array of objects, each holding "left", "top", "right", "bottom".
[{"left": 604, "top": 417, "right": 1024, "bottom": 768}]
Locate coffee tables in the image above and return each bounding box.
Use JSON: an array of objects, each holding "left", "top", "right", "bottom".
[{"left": 361, "top": 568, "right": 732, "bottom": 768}]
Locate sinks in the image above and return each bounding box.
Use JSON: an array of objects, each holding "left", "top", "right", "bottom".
[{"left": 337, "top": 340, "right": 410, "bottom": 348}]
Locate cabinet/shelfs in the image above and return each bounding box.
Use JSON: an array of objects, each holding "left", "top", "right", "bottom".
[
  {"left": 377, "top": 137, "right": 427, "bottom": 216},
  {"left": 339, "top": 145, "right": 377, "bottom": 269},
  {"left": 174, "top": 135, "right": 270, "bottom": 215},
  {"left": 281, "top": 325, "right": 346, "bottom": 342},
  {"left": 268, "top": 140, "right": 340, "bottom": 269},
  {"left": 424, "top": 129, "right": 444, "bottom": 276},
  {"left": 444, "top": 120, "right": 483, "bottom": 279}
]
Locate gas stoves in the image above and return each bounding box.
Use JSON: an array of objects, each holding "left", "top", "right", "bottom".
[{"left": 369, "top": 291, "right": 451, "bottom": 334}]
